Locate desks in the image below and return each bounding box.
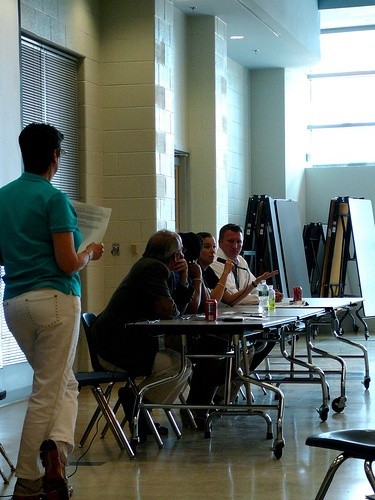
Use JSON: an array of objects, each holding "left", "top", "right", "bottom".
[
  {"left": 198, "top": 307, "right": 348, "bottom": 429},
  {"left": 260, "top": 298, "right": 371, "bottom": 399},
  {"left": 129, "top": 314, "right": 297, "bottom": 460}
]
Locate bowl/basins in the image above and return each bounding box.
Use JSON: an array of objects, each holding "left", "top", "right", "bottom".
[{"left": 274, "top": 289, "right": 283, "bottom": 302}]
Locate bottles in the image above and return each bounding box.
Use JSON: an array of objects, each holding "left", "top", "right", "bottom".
[
  {"left": 268, "top": 285, "right": 276, "bottom": 311},
  {"left": 258, "top": 279, "right": 269, "bottom": 314}
]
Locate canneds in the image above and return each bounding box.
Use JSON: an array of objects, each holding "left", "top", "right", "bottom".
[
  {"left": 293, "top": 286, "right": 303, "bottom": 302},
  {"left": 204, "top": 299, "right": 217, "bottom": 322}
]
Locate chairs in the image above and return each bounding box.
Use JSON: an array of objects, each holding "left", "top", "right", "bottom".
[
  {"left": 306, "top": 428, "right": 375, "bottom": 500},
  {"left": 75, "top": 369, "right": 137, "bottom": 460},
  {"left": 79, "top": 312, "right": 183, "bottom": 449}
]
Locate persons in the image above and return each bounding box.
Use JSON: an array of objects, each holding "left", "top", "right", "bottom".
[
  {"left": 0, "top": 120, "right": 109, "bottom": 500},
  {"left": 209, "top": 222, "right": 284, "bottom": 407},
  {"left": 196, "top": 232, "right": 278, "bottom": 412},
  {"left": 91, "top": 229, "right": 192, "bottom": 441},
  {"left": 176, "top": 231, "right": 216, "bottom": 421}
]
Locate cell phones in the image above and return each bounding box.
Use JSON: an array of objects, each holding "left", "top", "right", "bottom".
[{"left": 222, "top": 318, "right": 243, "bottom": 322}]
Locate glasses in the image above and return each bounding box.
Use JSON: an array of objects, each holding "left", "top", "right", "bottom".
[{"left": 60, "top": 149, "right": 67, "bottom": 158}]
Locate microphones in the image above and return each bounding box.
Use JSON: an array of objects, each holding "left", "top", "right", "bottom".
[{"left": 217, "top": 257, "right": 247, "bottom": 270}]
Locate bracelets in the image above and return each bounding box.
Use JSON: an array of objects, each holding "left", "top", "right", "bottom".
[
  {"left": 192, "top": 278, "right": 202, "bottom": 282},
  {"left": 252, "top": 281, "right": 257, "bottom": 287},
  {"left": 83, "top": 250, "right": 91, "bottom": 266},
  {"left": 217, "top": 283, "right": 225, "bottom": 288}
]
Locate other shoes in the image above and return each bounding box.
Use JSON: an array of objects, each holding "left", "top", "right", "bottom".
[
  {"left": 118, "top": 387, "right": 148, "bottom": 443},
  {"left": 13, "top": 478, "right": 73, "bottom": 500},
  {"left": 39, "top": 439, "right": 71, "bottom": 500}
]
